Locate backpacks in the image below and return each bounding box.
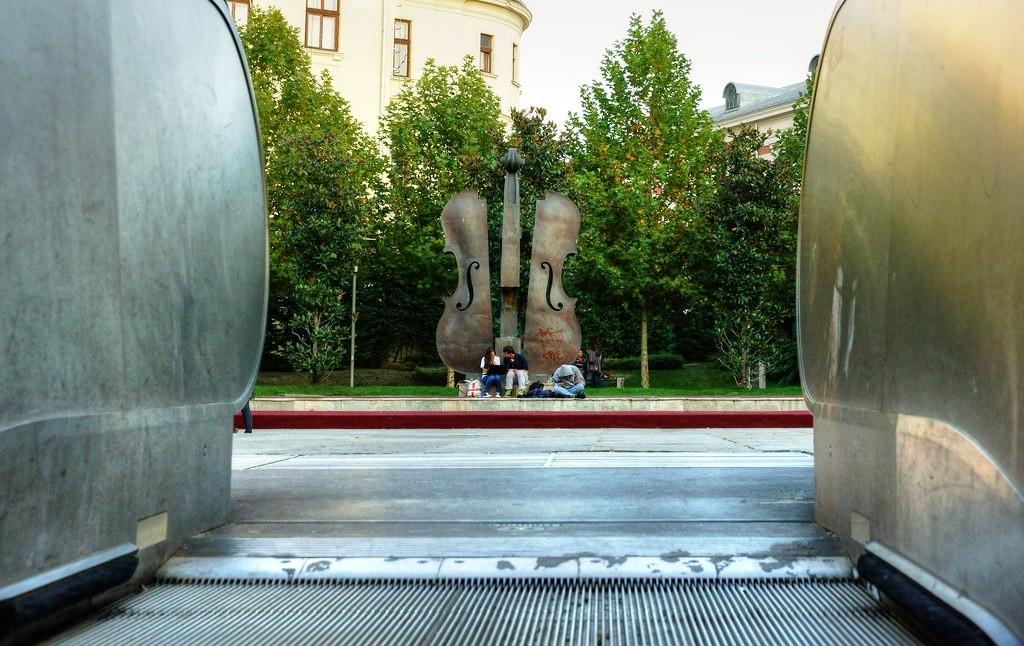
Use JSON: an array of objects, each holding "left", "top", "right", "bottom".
[{"left": 529, "top": 381, "right": 544, "bottom": 391}]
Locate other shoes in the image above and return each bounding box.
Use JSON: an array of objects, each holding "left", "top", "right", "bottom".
[
  {"left": 483, "top": 392, "right": 491, "bottom": 398},
  {"left": 516, "top": 389, "right": 525, "bottom": 397},
  {"left": 576, "top": 393, "right": 585, "bottom": 398},
  {"left": 553, "top": 392, "right": 564, "bottom": 397},
  {"left": 244, "top": 430, "right": 252, "bottom": 434},
  {"left": 503, "top": 390, "right": 513, "bottom": 397},
  {"left": 495, "top": 392, "right": 502, "bottom": 397}
]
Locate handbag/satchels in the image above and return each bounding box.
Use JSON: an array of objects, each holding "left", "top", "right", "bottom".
[{"left": 466, "top": 378, "right": 483, "bottom": 397}]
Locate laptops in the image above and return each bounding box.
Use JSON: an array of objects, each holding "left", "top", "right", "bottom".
[{"left": 484, "top": 365, "right": 508, "bottom": 375}]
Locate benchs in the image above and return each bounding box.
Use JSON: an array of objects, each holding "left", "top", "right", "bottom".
[
  {"left": 457, "top": 383, "right": 518, "bottom": 398},
  {"left": 585, "top": 377, "right": 624, "bottom": 388},
  {"left": 524, "top": 383, "right": 564, "bottom": 393}
]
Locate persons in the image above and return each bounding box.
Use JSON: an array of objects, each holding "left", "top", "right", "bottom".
[
  {"left": 551, "top": 364, "right": 586, "bottom": 398},
  {"left": 573, "top": 350, "right": 587, "bottom": 380},
  {"left": 480, "top": 347, "right": 502, "bottom": 397},
  {"left": 502, "top": 345, "right": 529, "bottom": 398},
  {"left": 586, "top": 348, "right": 604, "bottom": 388}
]
